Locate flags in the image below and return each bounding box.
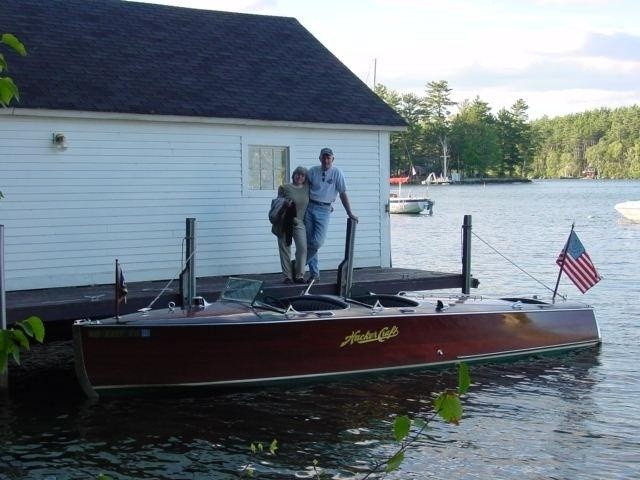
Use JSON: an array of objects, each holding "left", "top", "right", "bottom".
[
  {"left": 555, "top": 230, "right": 601, "bottom": 294},
  {"left": 117, "top": 265, "right": 128, "bottom": 305}
]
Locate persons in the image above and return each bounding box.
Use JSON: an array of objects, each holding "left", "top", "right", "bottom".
[
  {"left": 291, "top": 147, "right": 359, "bottom": 283},
  {"left": 270, "top": 166, "right": 334, "bottom": 284}
]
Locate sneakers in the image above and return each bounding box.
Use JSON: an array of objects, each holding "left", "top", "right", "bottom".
[{"left": 284, "top": 259, "right": 321, "bottom": 284}]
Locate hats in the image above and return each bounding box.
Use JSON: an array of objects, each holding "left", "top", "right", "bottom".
[{"left": 320, "top": 147, "right": 333, "bottom": 156}]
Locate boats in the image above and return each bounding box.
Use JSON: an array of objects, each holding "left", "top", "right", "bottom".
[
  {"left": 390, "top": 194, "right": 432, "bottom": 214},
  {"left": 421, "top": 177, "right": 452, "bottom": 185},
  {"left": 72, "top": 260, "right": 602, "bottom": 402}
]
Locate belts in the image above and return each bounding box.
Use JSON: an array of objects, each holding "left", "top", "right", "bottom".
[{"left": 309, "top": 198, "right": 331, "bottom": 207}]
